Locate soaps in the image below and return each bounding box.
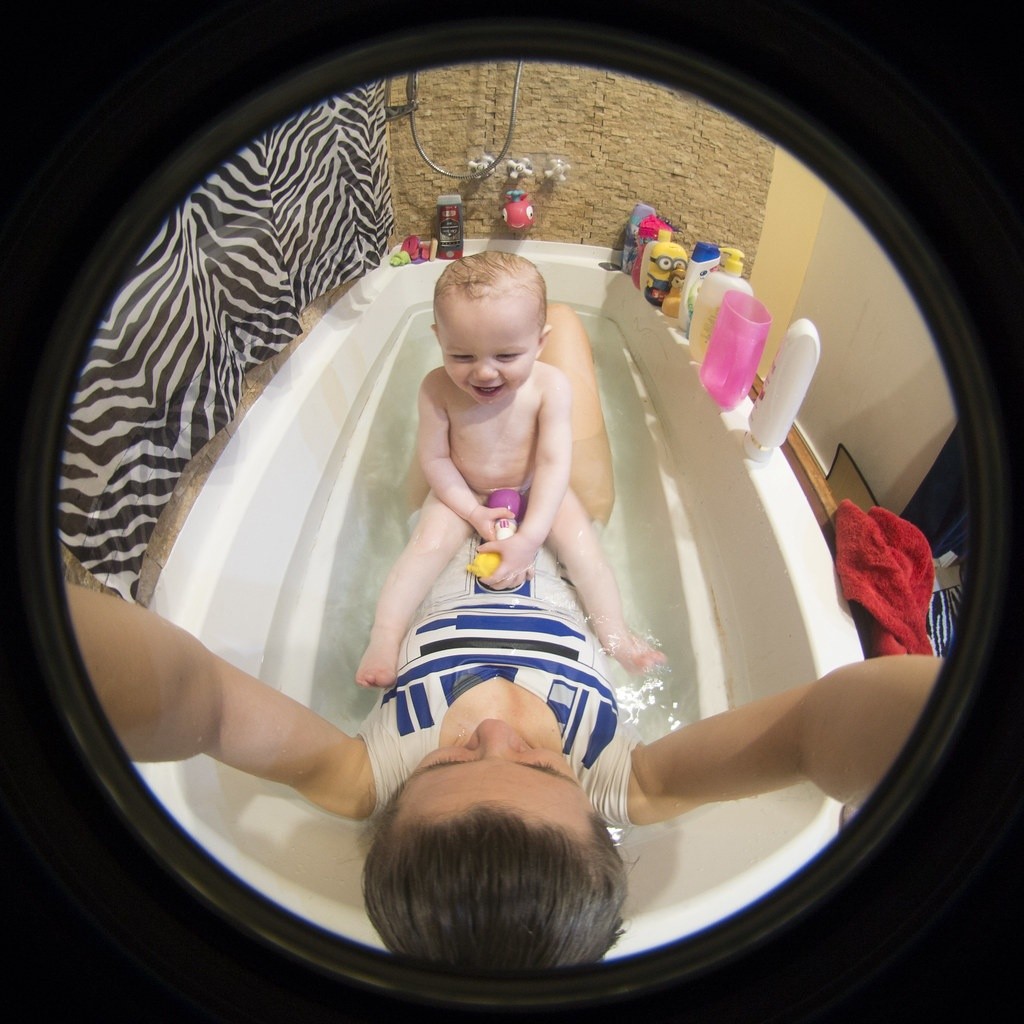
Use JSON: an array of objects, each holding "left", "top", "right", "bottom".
[{"left": 430, "top": 238, "right": 438, "bottom": 262}]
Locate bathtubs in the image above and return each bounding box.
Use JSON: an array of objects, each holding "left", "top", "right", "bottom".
[{"left": 132, "top": 240, "right": 866, "bottom": 967}]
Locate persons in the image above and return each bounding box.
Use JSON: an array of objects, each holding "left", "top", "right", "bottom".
[
  {"left": 64, "top": 301, "right": 943, "bottom": 970},
  {"left": 355, "top": 252, "right": 624, "bottom": 688}
]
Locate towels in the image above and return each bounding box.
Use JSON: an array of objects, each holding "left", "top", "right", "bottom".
[{"left": 835, "top": 499, "right": 934, "bottom": 657}]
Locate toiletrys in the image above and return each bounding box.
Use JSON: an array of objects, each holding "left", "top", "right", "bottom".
[
  {"left": 644, "top": 242, "right": 688, "bottom": 306},
  {"left": 689, "top": 248, "right": 753, "bottom": 364},
  {"left": 632, "top": 214, "right": 672, "bottom": 290},
  {"left": 678, "top": 242, "right": 721, "bottom": 332},
  {"left": 640, "top": 230, "right": 671, "bottom": 296},
  {"left": 743, "top": 319, "right": 821, "bottom": 463},
  {"left": 622, "top": 204, "right": 656, "bottom": 275}
]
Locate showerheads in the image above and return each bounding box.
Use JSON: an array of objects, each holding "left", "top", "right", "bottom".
[{"left": 407, "top": 70, "right": 420, "bottom": 105}]
